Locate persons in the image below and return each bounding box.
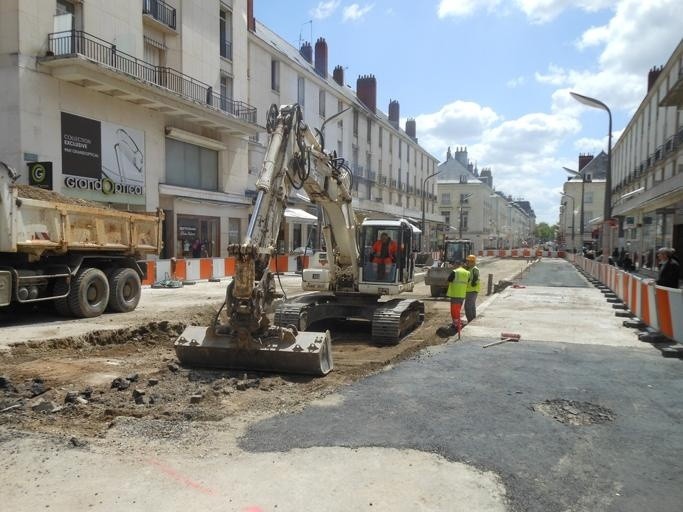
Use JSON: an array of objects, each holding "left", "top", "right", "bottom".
[
  {"left": 369, "top": 232, "right": 398, "bottom": 265},
  {"left": 542, "top": 244, "right": 681, "bottom": 289},
  {"left": 445, "top": 261, "right": 471, "bottom": 324},
  {"left": 199, "top": 244, "right": 208, "bottom": 258},
  {"left": 464, "top": 254, "right": 481, "bottom": 323}
]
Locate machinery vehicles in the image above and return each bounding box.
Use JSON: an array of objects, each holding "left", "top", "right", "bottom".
[
  {"left": 173, "top": 101, "right": 425, "bottom": 376},
  {"left": 424, "top": 237, "right": 475, "bottom": 295}
]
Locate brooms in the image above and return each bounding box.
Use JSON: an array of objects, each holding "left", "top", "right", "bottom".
[{"left": 482, "top": 332, "right": 521, "bottom": 347}]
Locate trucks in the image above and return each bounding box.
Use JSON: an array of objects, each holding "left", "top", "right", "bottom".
[{"left": 0, "top": 159, "right": 165, "bottom": 318}]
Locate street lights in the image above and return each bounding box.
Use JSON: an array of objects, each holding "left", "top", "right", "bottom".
[
  {"left": 459, "top": 193, "right": 474, "bottom": 239},
  {"left": 560, "top": 165, "right": 589, "bottom": 255},
  {"left": 570, "top": 91, "right": 614, "bottom": 260},
  {"left": 420, "top": 169, "right": 441, "bottom": 235},
  {"left": 323, "top": 106, "right": 354, "bottom": 215}
]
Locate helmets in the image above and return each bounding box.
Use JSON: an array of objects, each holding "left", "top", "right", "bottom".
[{"left": 466, "top": 254, "right": 476, "bottom": 260}]
